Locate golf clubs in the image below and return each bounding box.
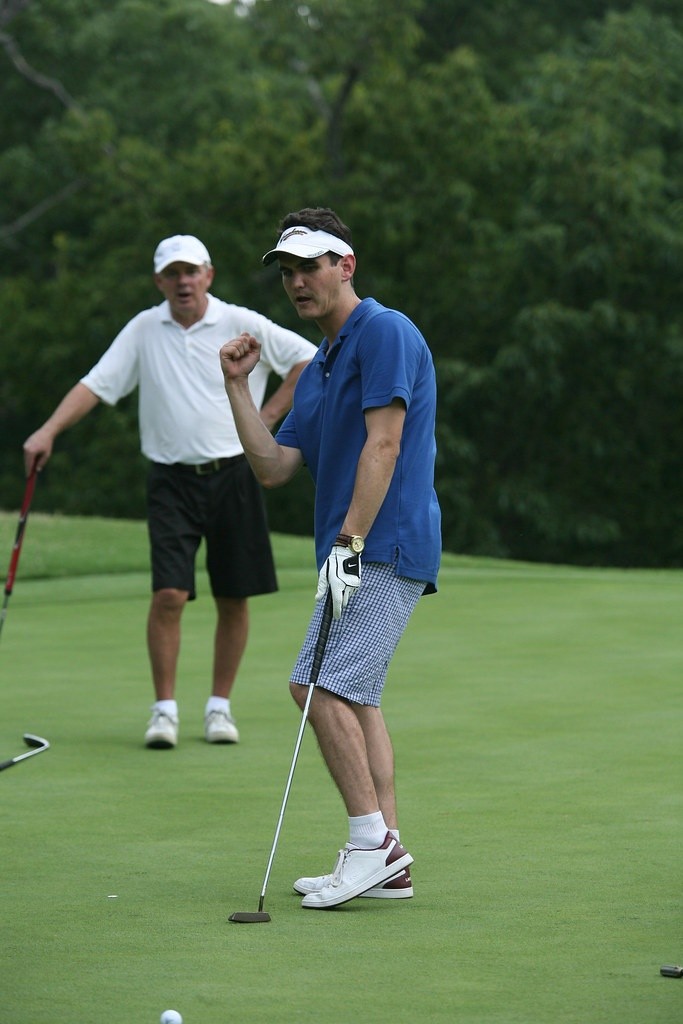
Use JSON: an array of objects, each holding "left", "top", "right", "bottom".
[
  {"left": 226, "top": 590, "right": 333, "bottom": 924},
  {"left": 0, "top": 732, "right": 53, "bottom": 768},
  {"left": 0, "top": 455, "right": 46, "bottom": 621}
]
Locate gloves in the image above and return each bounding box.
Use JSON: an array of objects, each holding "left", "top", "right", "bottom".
[{"left": 314, "top": 545, "right": 363, "bottom": 620}]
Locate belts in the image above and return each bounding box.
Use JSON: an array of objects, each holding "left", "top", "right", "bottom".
[{"left": 152, "top": 453, "right": 245, "bottom": 475}]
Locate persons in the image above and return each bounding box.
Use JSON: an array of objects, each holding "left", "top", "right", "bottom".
[
  {"left": 219, "top": 206, "right": 441, "bottom": 908},
  {"left": 21, "top": 233, "right": 320, "bottom": 749}
]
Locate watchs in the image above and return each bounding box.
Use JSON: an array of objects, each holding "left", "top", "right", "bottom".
[{"left": 335, "top": 533, "right": 364, "bottom": 553}]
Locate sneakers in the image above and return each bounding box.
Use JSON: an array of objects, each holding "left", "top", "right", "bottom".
[
  {"left": 144, "top": 699, "right": 179, "bottom": 747},
  {"left": 204, "top": 696, "right": 239, "bottom": 743},
  {"left": 293, "top": 831, "right": 414, "bottom": 909}
]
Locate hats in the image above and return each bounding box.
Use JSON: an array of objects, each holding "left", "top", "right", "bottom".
[
  {"left": 261, "top": 226, "right": 355, "bottom": 267},
  {"left": 153, "top": 235, "right": 211, "bottom": 274}
]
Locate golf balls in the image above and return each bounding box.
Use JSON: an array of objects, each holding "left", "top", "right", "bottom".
[{"left": 160, "top": 1009, "right": 181, "bottom": 1024}]
